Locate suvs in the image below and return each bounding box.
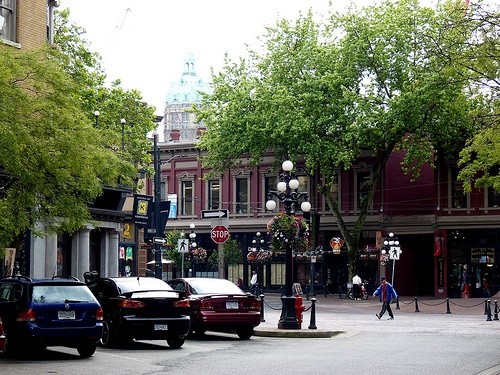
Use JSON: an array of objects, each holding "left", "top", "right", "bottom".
[{"left": 0, "top": 275, "right": 103, "bottom": 357}]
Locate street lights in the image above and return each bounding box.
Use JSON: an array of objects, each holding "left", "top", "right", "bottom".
[
  {"left": 149, "top": 115, "right": 164, "bottom": 280},
  {"left": 189, "top": 223, "right": 197, "bottom": 277},
  {"left": 94, "top": 110, "right": 100, "bottom": 126},
  {"left": 120, "top": 118, "right": 126, "bottom": 152},
  {"left": 382, "top": 233, "right": 402, "bottom": 286},
  {"left": 265, "top": 160, "right": 311, "bottom": 329},
  {"left": 252, "top": 231, "right": 265, "bottom": 295}
]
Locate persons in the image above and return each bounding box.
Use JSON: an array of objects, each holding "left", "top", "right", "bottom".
[
  {"left": 483, "top": 278, "right": 490, "bottom": 297},
  {"left": 458, "top": 269, "right": 471, "bottom": 291},
  {"left": 373, "top": 276, "right": 398, "bottom": 320},
  {"left": 236, "top": 278, "right": 242, "bottom": 286},
  {"left": 353, "top": 272, "right": 362, "bottom": 300},
  {"left": 250, "top": 271, "right": 257, "bottom": 295}
]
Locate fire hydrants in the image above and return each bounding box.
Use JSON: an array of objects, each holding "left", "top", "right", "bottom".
[{"left": 294, "top": 293, "right": 305, "bottom": 329}]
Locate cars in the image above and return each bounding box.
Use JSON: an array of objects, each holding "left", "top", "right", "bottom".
[
  {"left": 83, "top": 277, "right": 191, "bottom": 349},
  {"left": 167, "top": 277, "right": 262, "bottom": 339}
]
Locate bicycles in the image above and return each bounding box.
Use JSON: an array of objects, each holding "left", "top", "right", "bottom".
[{"left": 347, "top": 280, "right": 370, "bottom": 300}]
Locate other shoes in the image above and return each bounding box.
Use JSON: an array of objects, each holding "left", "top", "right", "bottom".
[
  {"left": 376, "top": 314, "right": 381, "bottom": 320},
  {"left": 387, "top": 317, "right": 394, "bottom": 320},
  {"left": 357, "top": 298, "right": 361, "bottom": 300},
  {"left": 355, "top": 298, "right": 356, "bottom": 300}
]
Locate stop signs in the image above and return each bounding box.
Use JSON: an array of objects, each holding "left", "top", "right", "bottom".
[{"left": 211, "top": 225, "right": 230, "bottom": 244}]
